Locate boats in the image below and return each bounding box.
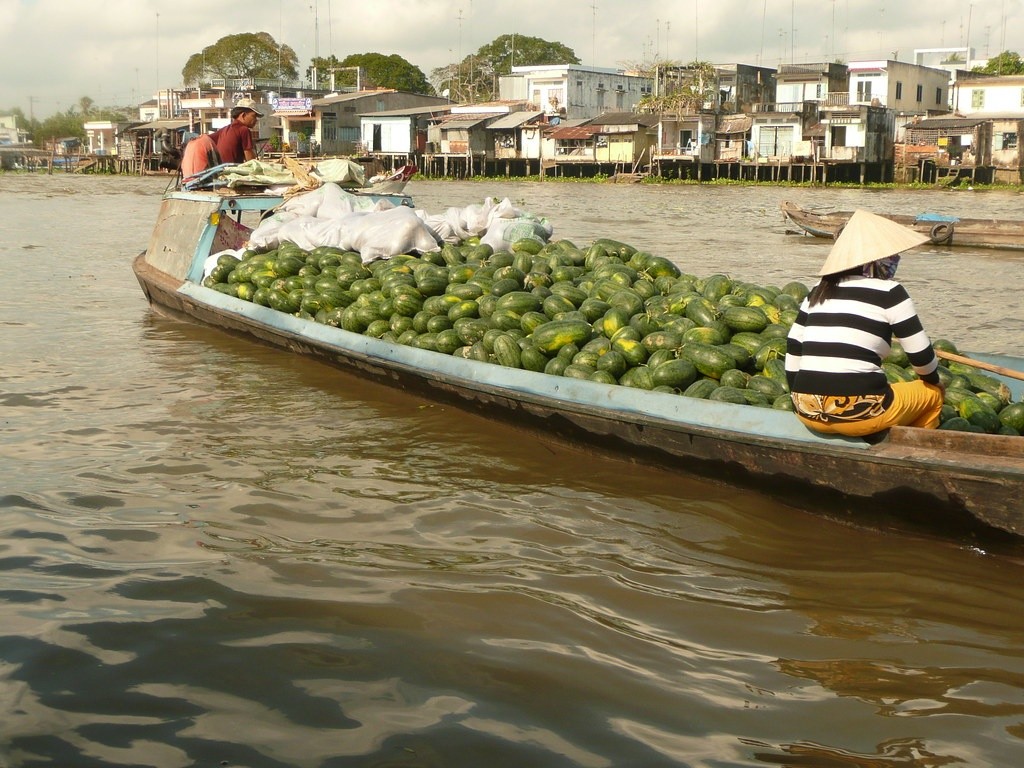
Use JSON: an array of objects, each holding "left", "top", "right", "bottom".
[
  {"left": 782, "top": 197, "right": 1024, "bottom": 253},
  {"left": 132, "top": 146, "right": 1024, "bottom": 546}
]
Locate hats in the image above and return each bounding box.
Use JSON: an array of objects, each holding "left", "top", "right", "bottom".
[
  {"left": 818, "top": 209, "right": 931, "bottom": 277},
  {"left": 236, "top": 98, "right": 264, "bottom": 118}
]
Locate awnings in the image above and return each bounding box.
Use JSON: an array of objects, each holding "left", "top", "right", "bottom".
[
  {"left": 716, "top": 117, "right": 753, "bottom": 133},
  {"left": 132, "top": 119, "right": 199, "bottom": 129},
  {"left": 801, "top": 121, "right": 826, "bottom": 138},
  {"left": 270, "top": 108, "right": 311, "bottom": 117},
  {"left": 440, "top": 120, "right": 483, "bottom": 130},
  {"left": 488, "top": 110, "right": 543, "bottom": 129},
  {"left": 548, "top": 124, "right": 599, "bottom": 140}
]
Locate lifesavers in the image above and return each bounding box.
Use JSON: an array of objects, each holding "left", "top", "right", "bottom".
[
  {"left": 833, "top": 222, "right": 848, "bottom": 244},
  {"left": 930, "top": 221, "right": 954, "bottom": 243}
]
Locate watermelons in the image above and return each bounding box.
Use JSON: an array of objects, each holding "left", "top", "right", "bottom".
[{"left": 203, "top": 236, "right": 1024, "bottom": 438}]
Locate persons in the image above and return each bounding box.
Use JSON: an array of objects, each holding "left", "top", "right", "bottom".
[
  {"left": 208, "top": 98, "right": 264, "bottom": 163},
  {"left": 786, "top": 209, "right": 944, "bottom": 436}
]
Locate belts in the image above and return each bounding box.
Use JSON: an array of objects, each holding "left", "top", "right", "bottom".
[{"left": 799, "top": 410, "right": 851, "bottom": 423}]
